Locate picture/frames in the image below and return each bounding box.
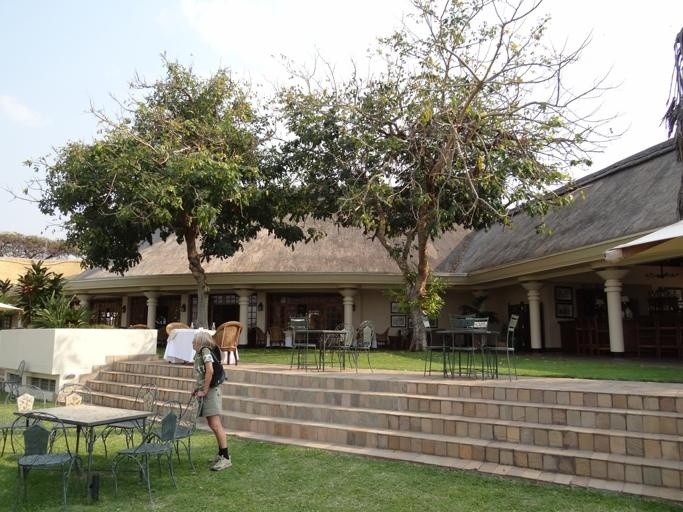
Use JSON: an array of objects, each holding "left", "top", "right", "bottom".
[
  {"left": 554, "top": 286, "right": 574, "bottom": 318},
  {"left": 391, "top": 301, "right": 438, "bottom": 328}
]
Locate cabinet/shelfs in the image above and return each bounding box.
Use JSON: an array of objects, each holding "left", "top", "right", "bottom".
[{"left": 508, "top": 301, "right": 545, "bottom": 352}]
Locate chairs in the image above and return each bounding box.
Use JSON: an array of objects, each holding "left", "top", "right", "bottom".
[
  {"left": 0, "top": 383, "right": 203, "bottom": 512},
  {"left": 255, "top": 326, "right": 285, "bottom": 349},
  {"left": 376, "top": 327, "right": 389, "bottom": 350},
  {"left": 165, "top": 321, "right": 244, "bottom": 366},
  {"left": 421, "top": 313, "right": 519, "bottom": 380},
  {"left": 0, "top": 360, "right": 25, "bottom": 403},
  {"left": 288, "top": 320, "right": 375, "bottom": 374}
]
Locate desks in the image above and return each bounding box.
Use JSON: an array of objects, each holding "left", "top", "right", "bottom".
[{"left": 283, "top": 330, "right": 347, "bottom": 372}]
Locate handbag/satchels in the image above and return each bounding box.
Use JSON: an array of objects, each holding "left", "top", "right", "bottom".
[{"left": 209, "top": 359, "right": 225, "bottom": 388}]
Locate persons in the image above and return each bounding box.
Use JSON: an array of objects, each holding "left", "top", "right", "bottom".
[{"left": 189, "top": 330, "right": 233, "bottom": 471}]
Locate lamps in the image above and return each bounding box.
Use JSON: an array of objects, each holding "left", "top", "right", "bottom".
[
  {"left": 181, "top": 304, "right": 186, "bottom": 312},
  {"left": 123, "top": 305, "right": 126, "bottom": 312},
  {"left": 258, "top": 302, "right": 263, "bottom": 311}
]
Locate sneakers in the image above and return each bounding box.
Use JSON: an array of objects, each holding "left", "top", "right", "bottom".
[{"left": 208, "top": 453, "right": 232, "bottom": 472}]
